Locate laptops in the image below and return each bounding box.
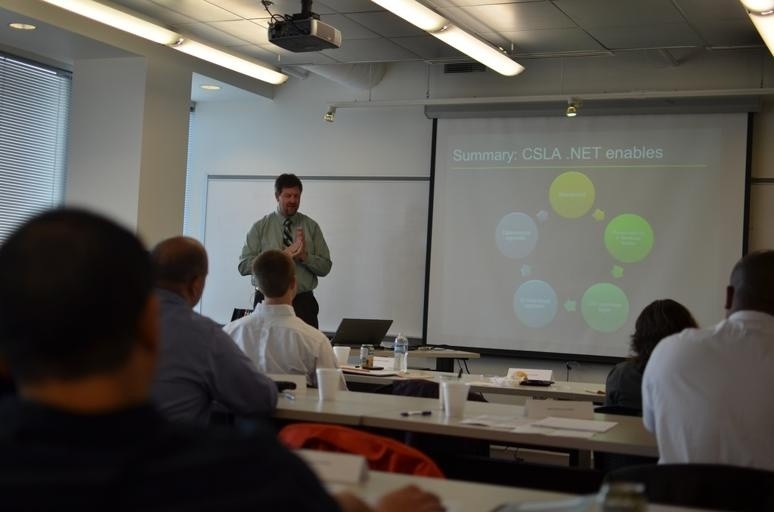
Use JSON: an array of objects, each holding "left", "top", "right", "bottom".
[{"left": 330, "top": 318, "right": 394, "bottom": 349}]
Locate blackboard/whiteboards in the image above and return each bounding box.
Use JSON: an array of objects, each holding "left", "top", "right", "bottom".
[
  {"left": 199, "top": 175, "right": 433, "bottom": 349},
  {"left": 748, "top": 178, "right": 774, "bottom": 252}
]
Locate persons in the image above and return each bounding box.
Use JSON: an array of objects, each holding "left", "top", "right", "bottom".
[
  {"left": 219, "top": 248, "right": 352, "bottom": 393},
  {"left": 135, "top": 234, "right": 280, "bottom": 444},
  {"left": 604, "top": 297, "right": 708, "bottom": 412},
  {"left": 1, "top": 208, "right": 451, "bottom": 512},
  {"left": 639, "top": 244, "right": 773, "bottom": 471},
  {"left": 236, "top": 172, "right": 334, "bottom": 328}
]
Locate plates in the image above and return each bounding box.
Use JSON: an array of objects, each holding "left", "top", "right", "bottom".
[{"left": 520, "top": 379, "right": 555, "bottom": 387}]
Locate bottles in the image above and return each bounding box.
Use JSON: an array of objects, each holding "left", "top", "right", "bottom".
[
  {"left": 394, "top": 332, "right": 408, "bottom": 372},
  {"left": 359, "top": 346, "right": 374, "bottom": 368}
]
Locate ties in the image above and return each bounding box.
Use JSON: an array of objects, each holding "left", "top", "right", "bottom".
[{"left": 282, "top": 218, "right": 293, "bottom": 252}]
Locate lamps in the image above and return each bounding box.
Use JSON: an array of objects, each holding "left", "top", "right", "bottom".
[
  {"left": 369, "top": 1, "right": 525, "bottom": 77},
  {"left": 324, "top": 93, "right": 583, "bottom": 122},
  {"left": 43, "top": 0, "right": 292, "bottom": 86},
  {"left": 743, "top": 0, "right": 774, "bottom": 56}
]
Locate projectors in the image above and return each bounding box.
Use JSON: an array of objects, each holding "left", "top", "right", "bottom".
[{"left": 268, "top": 18, "right": 342, "bottom": 53}]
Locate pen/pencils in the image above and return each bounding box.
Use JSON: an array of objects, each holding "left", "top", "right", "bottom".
[
  {"left": 285, "top": 393, "right": 295, "bottom": 401},
  {"left": 401, "top": 411, "right": 432, "bottom": 416}
]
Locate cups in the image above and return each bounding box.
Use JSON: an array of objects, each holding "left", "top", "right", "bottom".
[
  {"left": 443, "top": 381, "right": 471, "bottom": 421},
  {"left": 333, "top": 345, "right": 351, "bottom": 365},
  {"left": 312, "top": 366, "right": 342, "bottom": 402}
]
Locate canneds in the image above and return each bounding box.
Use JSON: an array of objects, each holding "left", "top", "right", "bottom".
[{"left": 359, "top": 344, "right": 374, "bottom": 369}]
